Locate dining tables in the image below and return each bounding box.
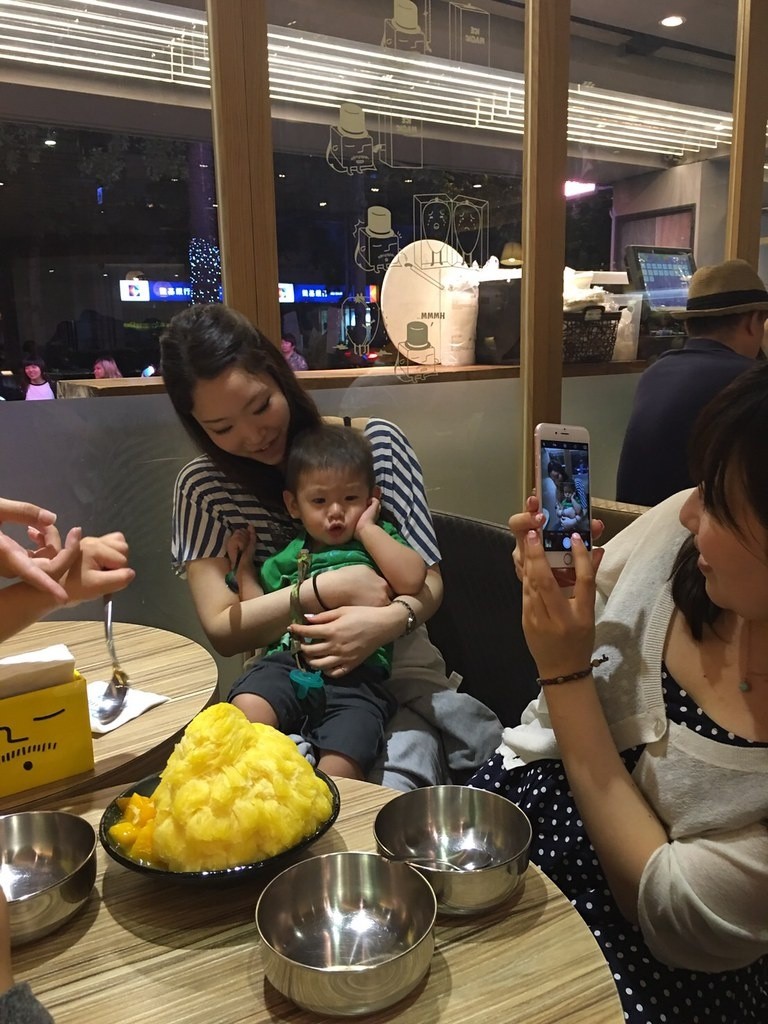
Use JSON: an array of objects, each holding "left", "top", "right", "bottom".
[{"left": 0, "top": 775, "right": 627, "bottom": 1024}]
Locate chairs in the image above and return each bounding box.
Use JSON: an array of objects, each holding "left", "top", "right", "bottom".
[{"left": 430, "top": 497, "right": 655, "bottom": 729}]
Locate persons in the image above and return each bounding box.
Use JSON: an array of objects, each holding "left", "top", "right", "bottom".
[
  {"left": 615, "top": 256, "right": 768, "bottom": 509},
  {"left": 223, "top": 424, "right": 427, "bottom": 783},
  {"left": 93, "top": 357, "right": 122, "bottom": 379},
  {"left": 280, "top": 333, "right": 308, "bottom": 371},
  {"left": 13, "top": 355, "right": 60, "bottom": 401},
  {"left": 555, "top": 479, "right": 581, "bottom": 518},
  {"left": 159, "top": 299, "right": 504, "bottom": 792},
  {"left": 461, "top": 362, "right": 768, "bottom": 1024},
  {"left": 0, "top": 493, "right": 136, "bottom": 646},
  {"left": 543, "top": 459, "right": 589, "bottom": 532}
]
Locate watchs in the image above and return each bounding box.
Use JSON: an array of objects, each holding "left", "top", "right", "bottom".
[{"left": 391, "top": 601, "right": 416, "bottom": 640}]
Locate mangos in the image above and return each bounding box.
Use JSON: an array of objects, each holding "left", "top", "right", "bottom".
[{"left": 107, "top": 793, "right": 158, "bottom": 862}]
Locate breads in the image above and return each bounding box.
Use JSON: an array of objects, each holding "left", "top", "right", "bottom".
[{"left": 149, "top": 702, "right": 333, "bottom": 872}]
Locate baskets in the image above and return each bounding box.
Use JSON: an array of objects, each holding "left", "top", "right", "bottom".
[{"left": 563, "top": 305, "right": 622, "bottom": 362}]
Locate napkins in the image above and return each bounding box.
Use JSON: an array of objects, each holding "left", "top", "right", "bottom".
[{"left": 87, "top": 680, "right": 170, "bottom": 734}]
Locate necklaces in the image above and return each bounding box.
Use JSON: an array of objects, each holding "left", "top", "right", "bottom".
[{"left": 739, "top": 619, "right": 768, "bottom": 694}]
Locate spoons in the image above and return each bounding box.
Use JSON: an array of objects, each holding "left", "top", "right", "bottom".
[{"left": 98, "top": 567, "right": 129, "bottom": 723}]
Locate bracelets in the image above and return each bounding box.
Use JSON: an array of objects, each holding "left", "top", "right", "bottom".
[
  {"left": 537, "top": 653, "right": 610, "bottom": 687},
  {"left": 313, "top": 570, "right": 330, "bottom": 611},
  {"left": 574, "top": 517, "right": 579, "bottom": 525}
]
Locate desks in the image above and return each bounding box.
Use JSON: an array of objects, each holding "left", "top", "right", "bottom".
[{"left": 0, "top": 621, "right": 220, "bottom": 814}]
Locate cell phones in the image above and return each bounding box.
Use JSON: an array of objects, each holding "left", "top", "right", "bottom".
[{"left": 535, "top": 423, "right": 592, "bottom": 567}]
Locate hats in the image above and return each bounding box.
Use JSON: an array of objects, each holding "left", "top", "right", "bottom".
[{"left": 669, "top": 259, "right": 768, "bottom": 320}]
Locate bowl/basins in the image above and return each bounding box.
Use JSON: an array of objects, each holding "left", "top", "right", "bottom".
[
  {"left": 372, "top": 785, "right": 532, "bottom": 918},
  {"left": 254, "top": 850, "right": 438, "bottom": 1016},
  {"left": 0, "top": 810, "right": 100, "bottom": 948},
  {"left": 96, "top": 765, "right": 342, "bottom": 888}
]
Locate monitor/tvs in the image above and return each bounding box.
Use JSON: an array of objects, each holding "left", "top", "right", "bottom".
[{"left": 624, "top": 243, "right": 698, "bottom": 317}]
